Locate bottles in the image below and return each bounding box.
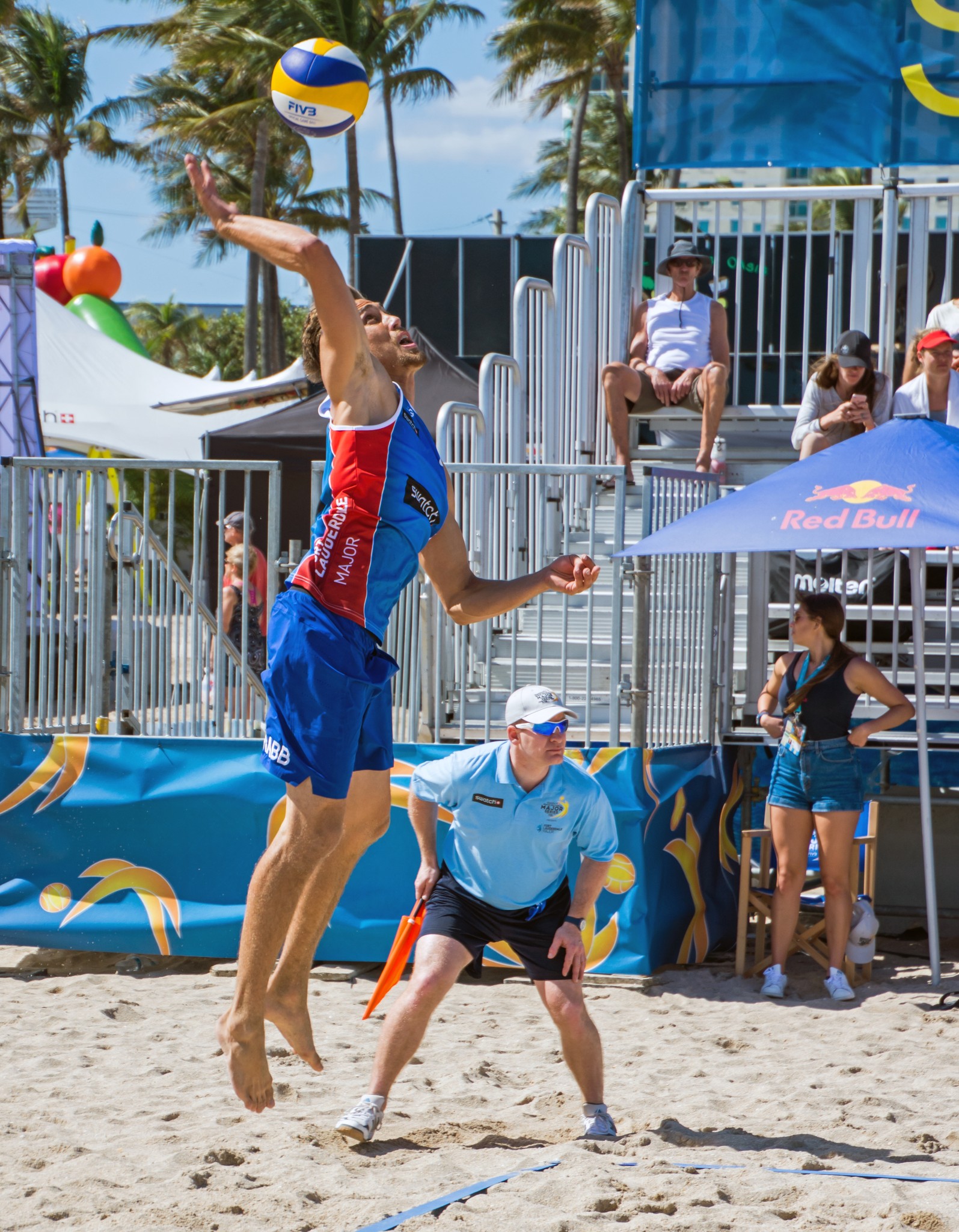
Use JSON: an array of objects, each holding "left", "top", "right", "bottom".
[
  {"left": 711, "top": 437, "right": 726, "bottom": 485},
  {"left": 116, "top": 957, "right": 151, "bottom": 972}
]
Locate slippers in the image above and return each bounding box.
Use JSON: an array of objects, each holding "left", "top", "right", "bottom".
[
  {"left": 694, "top": 480, "right": 709, "bottom": 487},
  {"left": 601, "top": 475, "right": 636, "bottom": 491}
]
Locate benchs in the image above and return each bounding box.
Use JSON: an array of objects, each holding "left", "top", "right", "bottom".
[{"left": 629, "top": 404, "right": 959, "bottom": 722}]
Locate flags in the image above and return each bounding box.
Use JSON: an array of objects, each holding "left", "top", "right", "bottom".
[{"left": 362, "top": 899, "right": 427, "bottom": 1019}]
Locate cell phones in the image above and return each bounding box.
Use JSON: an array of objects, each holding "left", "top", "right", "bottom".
[{"left": 849, "top": 394, "right": 867, "bottom": 410}]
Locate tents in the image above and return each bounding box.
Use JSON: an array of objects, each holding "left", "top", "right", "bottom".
[{"left": 35, "top": 287, "right": 306, "bottom": 479}]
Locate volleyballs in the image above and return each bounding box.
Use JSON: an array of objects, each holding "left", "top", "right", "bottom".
[{"left": 270, "top": 37, "right": 370, "bottom": 138}]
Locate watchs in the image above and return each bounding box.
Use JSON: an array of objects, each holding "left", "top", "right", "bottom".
[
  {"left": 564, "top": 915, "right": 586, "bottom": 931},
  {"left": 756, "top": 713, "right": 768, "bottom": 725}
]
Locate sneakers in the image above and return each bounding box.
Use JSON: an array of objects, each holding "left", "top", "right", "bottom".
[
  {"left": 824, "top": 966, "right": 854, "bottom": 1001},
  {"left": 581, "top": 1100, "right": 617, "bottom": 1139},
  {"left": 760, "top": 964, "right": 787, "bottom": 997},
  {"left": 335, "top": 1094, "right": 385, "bottom": 1142}
]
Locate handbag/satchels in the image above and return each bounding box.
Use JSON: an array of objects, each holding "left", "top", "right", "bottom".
[{"left": 200, "top": 672, "right": 214, "bottom": 706}]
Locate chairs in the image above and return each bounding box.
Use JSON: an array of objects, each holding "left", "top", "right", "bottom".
[{"left": 736, "top": 801, "right": 880, "bottom": 988}]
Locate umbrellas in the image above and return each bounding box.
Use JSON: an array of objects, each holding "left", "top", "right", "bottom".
[{"left": 613, "top": 411, "right": 959, "bottom": 988}]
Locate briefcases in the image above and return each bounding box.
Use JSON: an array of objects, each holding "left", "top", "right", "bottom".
[{"left": 770, "top": 549, "right": 912, "bottom": 644}]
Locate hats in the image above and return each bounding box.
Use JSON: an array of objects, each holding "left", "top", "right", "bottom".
[
  {"left": 656, "top": 240, "right": 712, "bottom": 277},
  {"left": 216, "top": 511, "right": 253, "bottom": 531},
  {"left": 833, "top": 330, "right": 872, "bottom": 368},
  {"left": 917, "top": 330, "right": 958, "bottom": 349},
  {"left": 505, "top": 684, "right": 577, "bottom": 726},
  {"left": 845, "top": 900, "right": 879, "bottom": 963}
]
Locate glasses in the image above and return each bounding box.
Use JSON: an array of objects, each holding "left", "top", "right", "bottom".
[
  {"left": 514, "top": 719, "right": 569, "bottom": 736},
  {"left": 669, "top": 257, "right": 699, "bottom": 267},
  {"left": 224, "top": 558, "right": 228, "bottom": 563}
]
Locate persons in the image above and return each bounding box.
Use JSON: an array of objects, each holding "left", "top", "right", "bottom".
[
  {"left": 755, "top": 593, "right": 916, "bottom": 1000},
  {"left": 183, "top": 152, "right": 602, "bottom": 1112},
  {"left": 791, "top": 297, "right": 959, "bottom": 551},
  {"left": 336, "top": 682, "right": 617, "bottom": 1142},
  {"left": 106, "top": 502, "right": 267, "bottom": 718},
  {"left": 600, "top": 240, "right": 732, "bottom": 486}
]
